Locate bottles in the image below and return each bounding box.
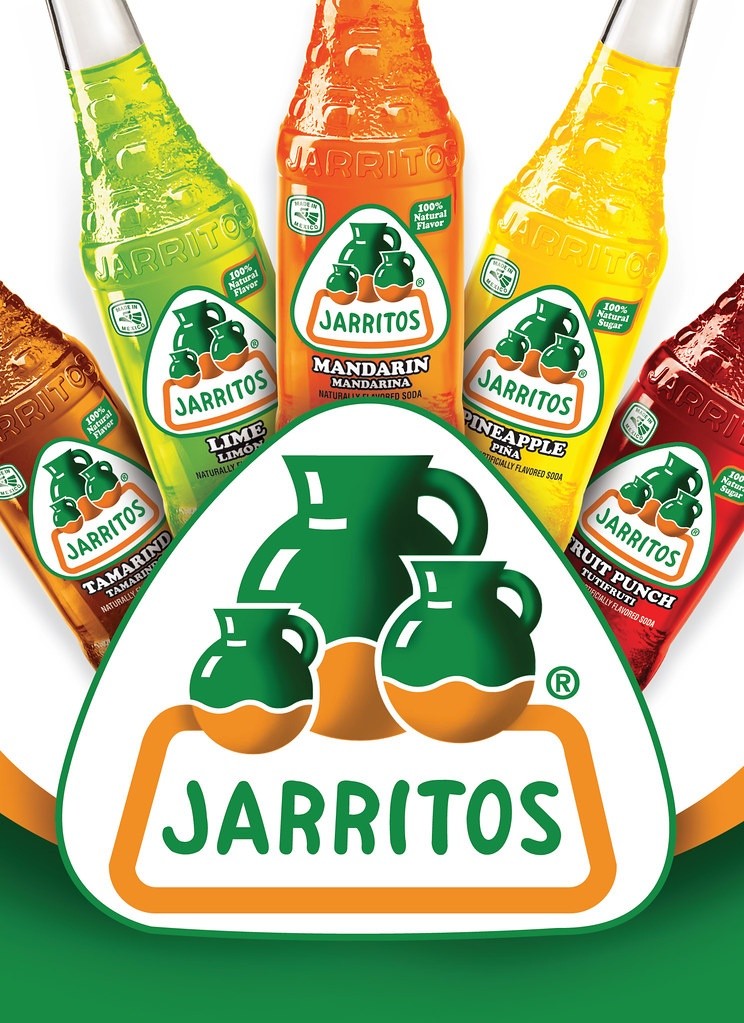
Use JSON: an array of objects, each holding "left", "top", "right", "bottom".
[
  {"left": 45, "top": 0, "right": 277, "bottom": 539},
  {"left": 0, "top": 283, "right": 171, "bottom": 672},
  {"left": 564, "top": 270, "right": 744, "bottom": 693},
  {"left": 463, "top": 0, "right": 697, "bottom": 557},
  {"left": 278, "top": 0, "right": 464, "bottom": 439}
]
